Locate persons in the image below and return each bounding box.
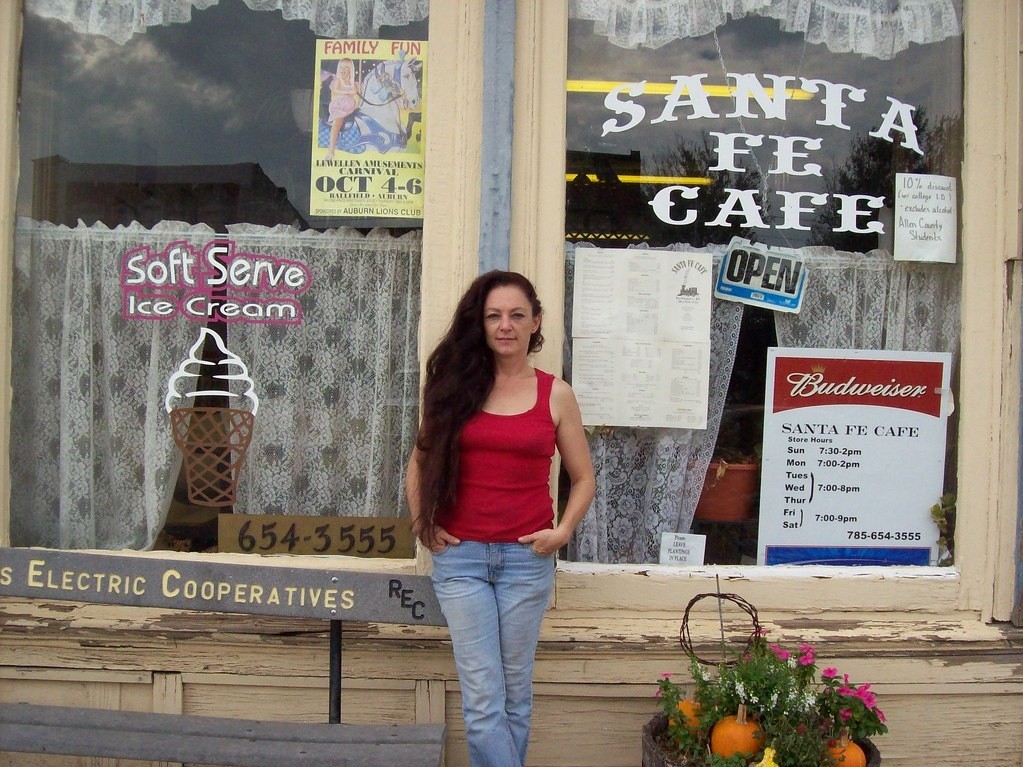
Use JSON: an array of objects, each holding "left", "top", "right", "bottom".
[
  {"left": 405, "top": 269, "right": 595, "bottom": 767},
  {"left": 325, "top": 57, "right": 359, "bottom": 161}
]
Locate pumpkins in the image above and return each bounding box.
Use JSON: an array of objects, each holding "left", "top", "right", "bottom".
[
  {"left": 710, "top": 703, "right": 765, "bottom": 759},
  {"left": 824, "top": 727, "right": 866, "bottom": 767},
  {"left": 668, "top": 697, "right": 703, "bottom": 743}
]
